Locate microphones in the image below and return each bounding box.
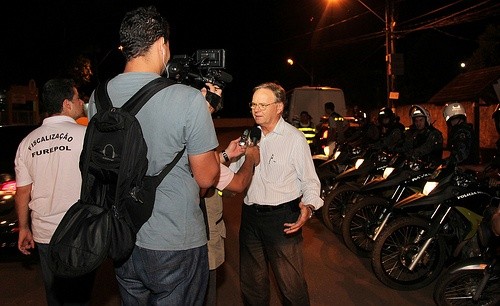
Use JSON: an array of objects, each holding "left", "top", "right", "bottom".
[{"left": 250, "top": 127, "right": 261, "bottom": 144}]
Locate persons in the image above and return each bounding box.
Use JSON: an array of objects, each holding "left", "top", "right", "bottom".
[
  {"left": 194, "top": 138, "right": 259, "bottom": 305},
  {"left": 14, "top": 74, "right": 90, "bottom": 306},
  {"left": 224, "top": 82, "right": 324, "bottom": 306},
  {"left": 85, "top": 7, "right": 220, "bottom": 306},
  {"left": 295, "top": 100, "right": 475, "bottom": 189}
]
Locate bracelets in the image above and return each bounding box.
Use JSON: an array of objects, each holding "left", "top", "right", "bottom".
[{"left": 306, "top": 205, "right": 313, "bottom": 220}]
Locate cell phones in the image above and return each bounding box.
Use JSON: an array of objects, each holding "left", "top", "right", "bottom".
[{"left": 240, "top": 128, "right": 249, "bottom": 143}]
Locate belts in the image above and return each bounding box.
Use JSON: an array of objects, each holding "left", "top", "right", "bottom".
[{"left": 242, "top": 198, "right": 300, "bottom": 213}]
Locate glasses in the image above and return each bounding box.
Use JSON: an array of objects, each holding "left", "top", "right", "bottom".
[{"left": 248, "top": 100, "right": 280, "bottom": 110}]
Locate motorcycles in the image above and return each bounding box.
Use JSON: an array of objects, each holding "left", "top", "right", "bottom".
[{"left": 311, "top": 118, "right": 499, "bottom": 306}]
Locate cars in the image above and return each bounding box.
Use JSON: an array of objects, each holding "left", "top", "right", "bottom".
[{"left": 1, "top": 125, "right": 44, "bottom": 250}]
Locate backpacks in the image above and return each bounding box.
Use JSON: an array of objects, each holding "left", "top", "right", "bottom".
[{"left": 46, "top": 77, "right": 186, "bottom": 277}]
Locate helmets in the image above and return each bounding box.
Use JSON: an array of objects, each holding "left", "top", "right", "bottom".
[
  {"left": 443, "top": 102, "right": 466, "bottom": 123},
  {"left": 409, "top": 105, "right": 431, "bottom": 127},
  {"left": 353, "top": 104, "right": 369, "bottom": 124},
  {"left": 378, "top": 107, "right": 393, "bottom": 127}
]
